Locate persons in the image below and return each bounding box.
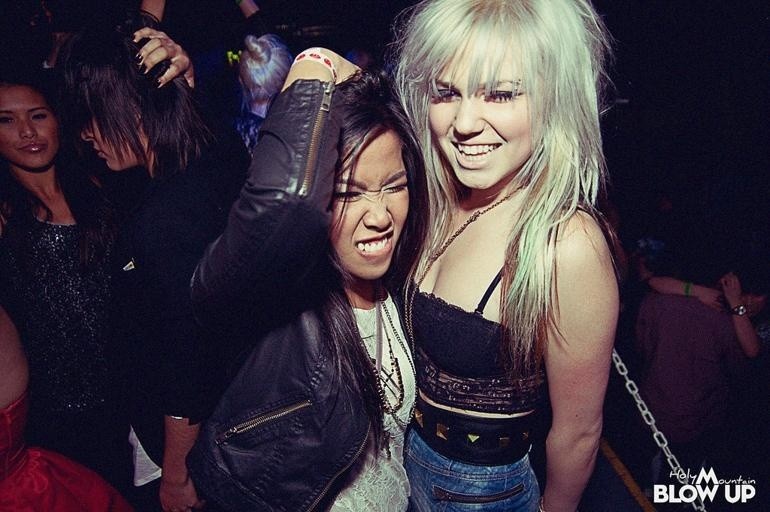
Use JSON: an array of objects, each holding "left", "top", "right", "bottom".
[{"left": 0, "top": 1, "right": 769, "bottom": 512}]
